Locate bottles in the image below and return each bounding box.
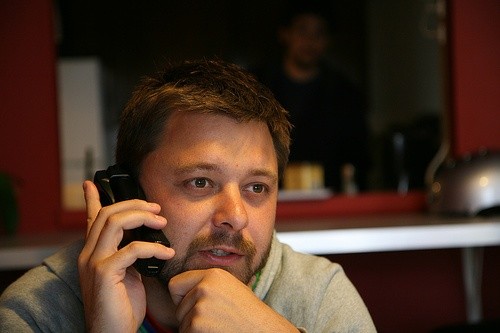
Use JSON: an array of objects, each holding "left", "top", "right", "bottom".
[{"left": 338, "top": 165, "right": 357, "bottom": 195}]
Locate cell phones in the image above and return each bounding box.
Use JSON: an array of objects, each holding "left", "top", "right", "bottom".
[{"left": 94, "top": 165, "right": 170, "bottom": 275}]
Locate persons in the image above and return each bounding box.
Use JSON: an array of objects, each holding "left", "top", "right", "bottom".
[
  {"left": 236, "top": 6, "right": 367, "bottom": 196},
  {"left": 0, "top": 58, "right": 378, "bottom": 332}
]
